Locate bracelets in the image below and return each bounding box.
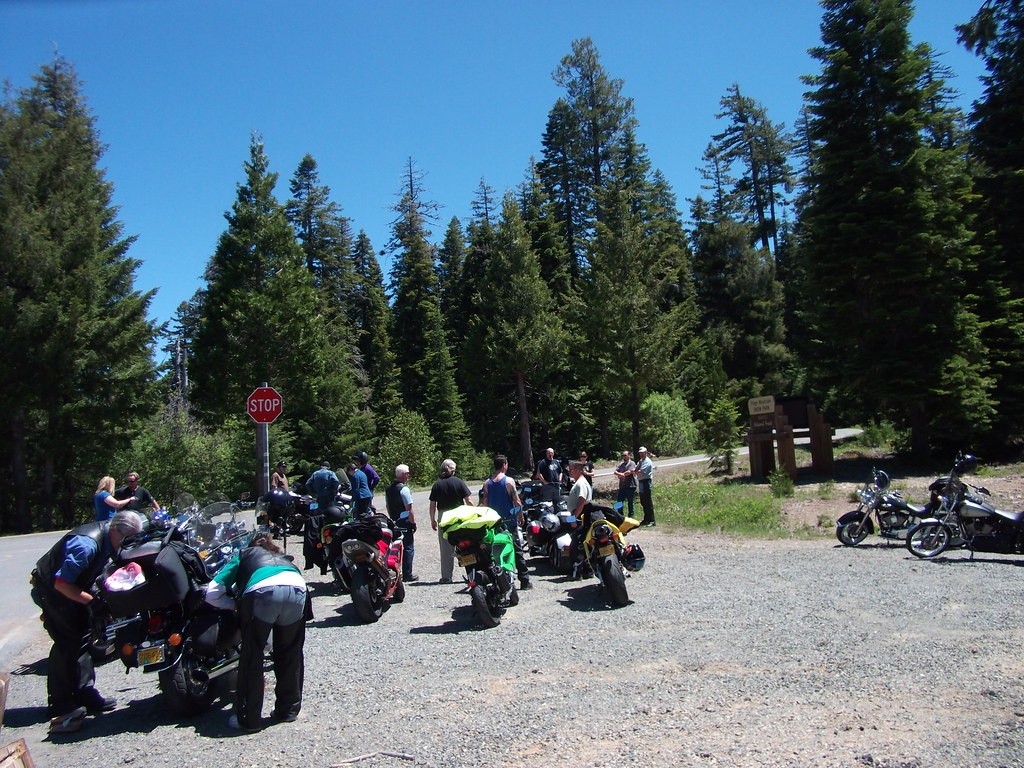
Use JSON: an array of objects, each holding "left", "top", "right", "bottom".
[{"left": 520, "top": 515, "right": 524, "bottom": 519}]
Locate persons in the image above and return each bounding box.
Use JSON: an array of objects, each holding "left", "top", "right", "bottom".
[
  {"left": 386, "top": 464, "right": 419, "bottom": 582},
  {"left": 347, "top": 464, "right": 373, "bottom": 515},
  {"left": 305, "top": 461, "right": 340, "bottom": 509},
  {"left": 94, "top": 476, "right": 135, "bottom": 522},
  {"left": 483, "top": 455, "right": 533, "bottom": 589},
  {"left": 30, "top": 511, "right": 143, "bottom": 721},
  {"left": 581, "top": 451, "right": 595, "bottom": 487},
  {"left": 271, "top": 460, "right": 290, "bottom": 540},
  {"left": 429, "top": 459, "right": 474, "bottom": 583},
  {"left": 631, "top": 446, "right": 656, "bottom": 527},
  {"left": 356, "top": 451, "right": 380, "bottom": 492},
  {"left": 566, "top": 461, "right": 592, "bottom": 530},
  {"left": 614, "top": 451, "right": 638, "bottom": 517},
  {"left": 205, "top": 535, "right": 314, "bottom": 731},
  {"left": 114, "top": 472, "right": 160, "bottom": 513},
  {"left": 533, "top": 448, "right": 572, "bottom": 490}
]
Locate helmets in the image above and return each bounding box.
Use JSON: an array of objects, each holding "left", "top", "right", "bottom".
[
  {"left": 875, "top": 470, "right": 890, "bottom": 491},
  {"left": 260, "top": 488, "right": 292, "bottom": 523},
  {"left": 358, "top": 451, "right": 368, "bottom": 464},
  {"left": 957, "top": 454, "right": 977, "bottom": 477},
  {"left": 621, "top": 545, "right": 645, "bottom": 572}
]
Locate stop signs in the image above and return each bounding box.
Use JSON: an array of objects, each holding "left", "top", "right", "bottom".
[{"left": 245, "top": 387, "right": 282, "bottom": 425}]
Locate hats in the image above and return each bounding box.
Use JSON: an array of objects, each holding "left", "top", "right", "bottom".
[
  {"left": 638, "top": 446, "right": 647, "bottom": 452},
  {"left": 276, "top": 461, "right": 287, "bottom": 467}
]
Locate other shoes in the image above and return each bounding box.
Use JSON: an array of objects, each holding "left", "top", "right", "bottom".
[
  {"left": 273, "top": 533, "right": 282, "bottom": 540},
  {"left": 440, "top": 578, "right": 452, "bottom": 583},
  {"left": 271, "top": 709, "right": 297, "bottom": 721},
  {"left": 228, "top": 715, "right": 262, "bottom": 732},
  {"left": 640, "top": 520, "right": 655, "bottom": 526},
  {"left": 521, "top": 582, "right": 532, "bottom": 590},
  {"left": 403, "top": 576, "right": 419, "bottom": 581},
  {"left": 77, "top": 700, "right": 117, "bottom": 711},
  {"left": 560, "top": 575, "right": 582, "bottom": 582},
  {"left": 281, "top": 533, "right": 290, "bottom": 537},
  {"left": 51, "top": 705, "right": 87, "bottom": 724}
]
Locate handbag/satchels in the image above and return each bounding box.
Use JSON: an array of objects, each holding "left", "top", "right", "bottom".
[
  {"left": 333, "top": 520, "right": 382, "bottom": 544},
  {"left": 97, "top": 526, "right": 188, "bottom": 609}
]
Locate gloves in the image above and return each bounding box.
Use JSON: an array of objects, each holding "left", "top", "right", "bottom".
[{"left": 87, "top": 595, "right": 112, "bottom": 641}]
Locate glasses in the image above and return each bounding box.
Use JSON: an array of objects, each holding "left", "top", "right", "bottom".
[
  {"left": 128, "top": 480, "right": 136, "bottom": 483},
  {"left": 580, "top": 455, "right": 586, "bottom": 458}
]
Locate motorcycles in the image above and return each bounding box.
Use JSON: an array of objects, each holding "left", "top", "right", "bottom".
[
  {"left": 905, "top": 450, "right": 1024, "bottom": 562},
  {"left": 564, "top": 499, "right": 631, "bottom": 607},
  {"left": 447, "top": 503, "right": 524, "bottom": 626},
  {"left": 316, "top": 505, "right": 410, "bottom": 623},
  {"left": 833, "top": 461, "right": 951, "bottom": 545},
  {"left": 81, "top": 492, "right": 266, "bottom": 723},
  {"left": 255, "top": 487, "right": 351, "bottom": 595},
  {"left": 508, "top": 475, "right": 580, "bottom": 575}
]
